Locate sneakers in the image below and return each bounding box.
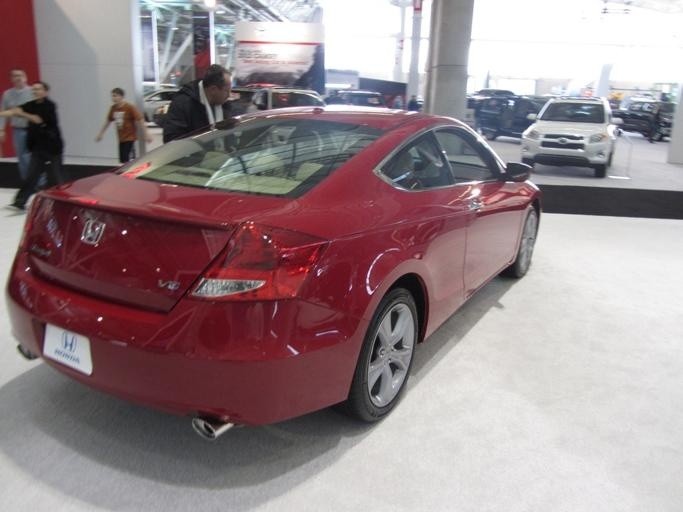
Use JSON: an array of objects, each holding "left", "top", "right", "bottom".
[{"left": 7, "top": 203, "right": 26, "bottom": 212}]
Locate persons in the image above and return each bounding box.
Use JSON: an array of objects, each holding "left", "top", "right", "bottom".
[
  {"left": 323, "top": 89, "right": 345, "bottom": 104},
  {"left": 0, "top": 81, "right": 64, "bottom": 213},
  {"left": 406, "top": 94, "right": 421, "bottom": 112},
  {"left": 0, "top": 68, "right": 47, "bottom": 196},
  {"left": 161, "top": 64, "right": 239, "bottom": 167},
  {"left": 390, "top": 96, "right": 403, "bottom": 109},
  {"left": 92, "top": 87, "right": 152, "bottom": 165}
]
